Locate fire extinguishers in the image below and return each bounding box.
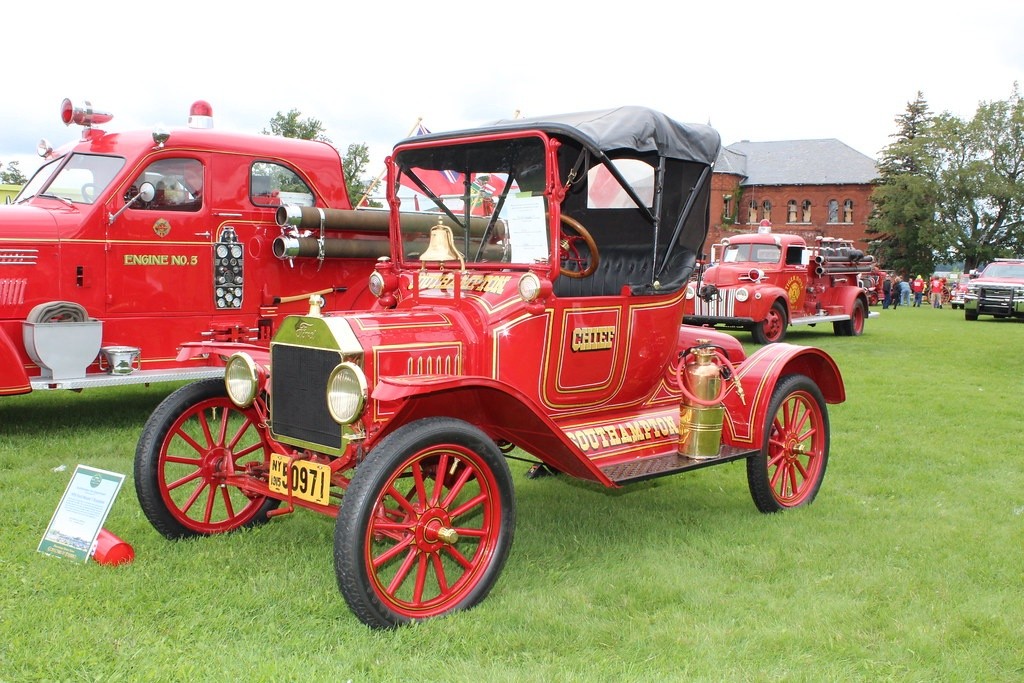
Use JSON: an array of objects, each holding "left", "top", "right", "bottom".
[{"left": 675, "top": 337, "right": 747, "bottom": 459}]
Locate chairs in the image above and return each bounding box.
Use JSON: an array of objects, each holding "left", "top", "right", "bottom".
[{"left": 148, "top": 177, "right": 187, "bottom": 210}]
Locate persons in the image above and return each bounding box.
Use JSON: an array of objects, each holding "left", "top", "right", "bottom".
[{"left": 882, "top": 274, "right": 958, "bottom": 309}]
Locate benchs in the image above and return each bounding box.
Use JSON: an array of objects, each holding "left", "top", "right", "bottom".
[{"left": 549, "top": 246, "right": 694, "bottom": 297}]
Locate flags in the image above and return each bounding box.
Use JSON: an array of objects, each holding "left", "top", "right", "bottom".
[{"left": 386, "top": 123, "right": 519, "bottom": 218}]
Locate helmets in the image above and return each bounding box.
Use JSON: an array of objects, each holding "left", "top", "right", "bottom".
[
  {"left": 935, "top": 276, "right": 939, "bottom": 279},
  {"left": 917, "top": 275, "right": 921, "bottom": 279}
]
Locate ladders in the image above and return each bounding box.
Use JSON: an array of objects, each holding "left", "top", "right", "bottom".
[{"left": 814, "top": 239, "right": 855, "bottom": 256}]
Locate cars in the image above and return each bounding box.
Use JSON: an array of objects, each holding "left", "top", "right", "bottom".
[{"left": 130, "top": 107, "right": 845, "bottom": 632}]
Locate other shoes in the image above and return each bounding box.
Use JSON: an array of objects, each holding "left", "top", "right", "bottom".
[
  {"left": 913, "top": 304, "right": 915, "bottom": 307},
  {"left": 917, "top": 305, "right": 920, "bottom": 307},
  {"left": 940, "top": 305, "right": 943, "bottom": 309}
]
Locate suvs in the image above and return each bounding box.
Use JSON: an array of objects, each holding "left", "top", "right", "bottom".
[
  {"left": 949, "top": 269, "right": 983, "bottom": 309},
  {"left": 962, "top": 258, "right": 1024, "bottom": 320},
  {"left": 857, "top": 270, "right": 896, "bottom": 307}
]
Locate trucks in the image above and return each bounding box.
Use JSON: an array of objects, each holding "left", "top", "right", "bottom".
[
  {"left": 683, "top": 220, "right": 877, "bottom": 348},
  {"left": 0, "top": 96, "right": 511, "bottom": 398}
]
